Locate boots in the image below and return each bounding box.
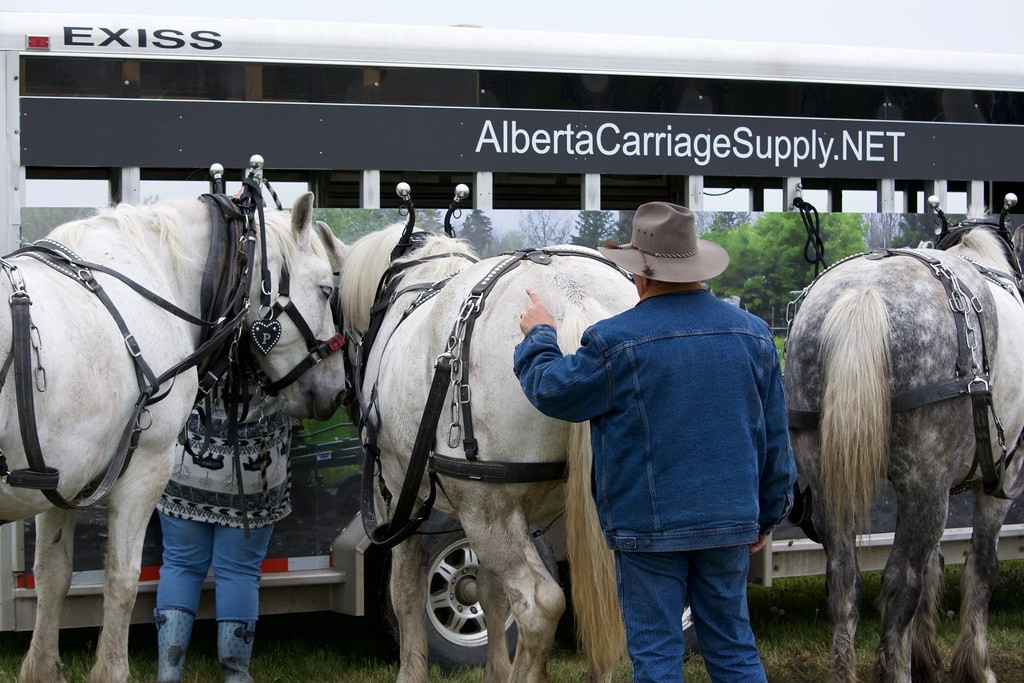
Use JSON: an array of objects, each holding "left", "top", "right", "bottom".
[
  {"left": 153, "top": 606, "right": 196, "bottom": 683},
  {"left": 216, "top": 617, "right": 258, "bottom": 683}
]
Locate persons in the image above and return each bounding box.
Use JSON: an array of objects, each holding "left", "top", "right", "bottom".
[
  {"left": 153, "top": 381, "right": 294, "bottom": 682},
  {"left": 513, "top": 202, "right": 796, "bottom": 683}
]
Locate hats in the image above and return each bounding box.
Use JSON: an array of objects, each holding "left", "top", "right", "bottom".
[{"left": 598, "top": 202, "right": 730, "bottom": 283}]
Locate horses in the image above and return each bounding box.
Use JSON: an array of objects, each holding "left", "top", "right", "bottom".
[
  {"left": 783, "top": 224, "right": 1024, "bottom": 683},
  {"left": 314, "top": 212, "right": 642, "bottom": 682},
  {"left": 0, "top": 191, "right": 347, "bottom": 682}
]
